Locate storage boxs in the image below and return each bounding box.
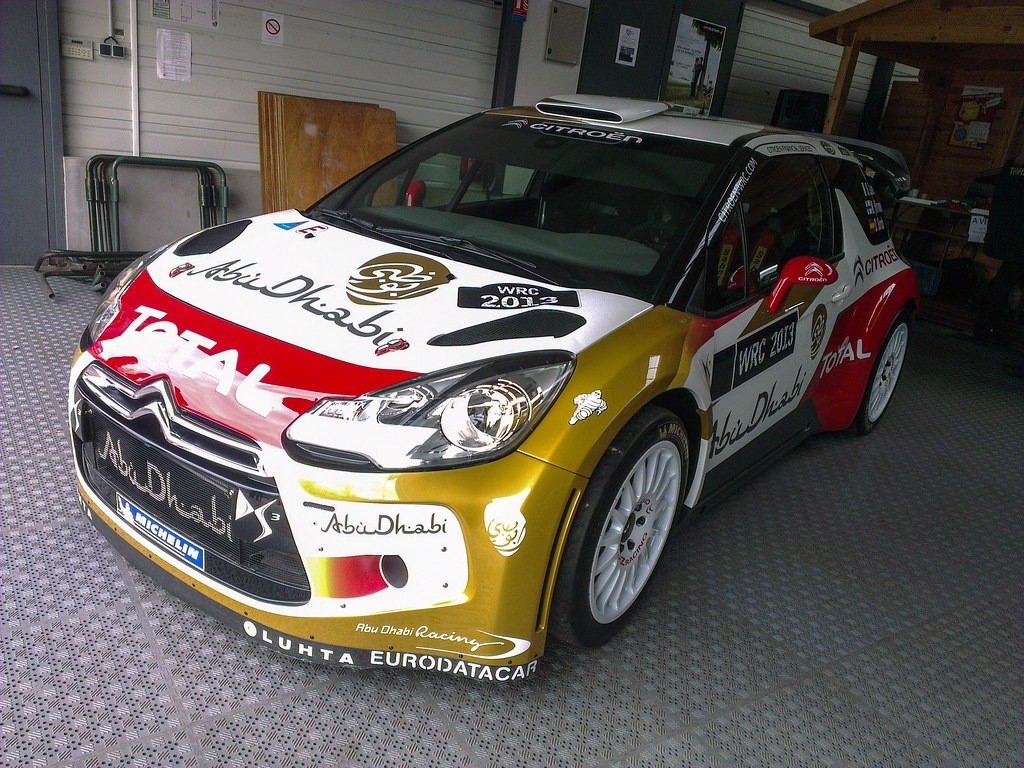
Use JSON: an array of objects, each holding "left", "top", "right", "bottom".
[{"left": 889, "top": 204, "right": 924, "bottom": 225}]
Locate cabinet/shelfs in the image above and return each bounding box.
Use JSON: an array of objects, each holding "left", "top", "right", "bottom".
[{"left": 888, "top": 197, "right": 1024, "bottom": 351}]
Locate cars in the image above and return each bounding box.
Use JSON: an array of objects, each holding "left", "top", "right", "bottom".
[{"left": 67, "top": 94, "right": 922, "bottom": 684}]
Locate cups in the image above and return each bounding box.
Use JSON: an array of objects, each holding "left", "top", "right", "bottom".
[{"left": 911, "top": 189, "right": 919, "bottom": 198}]
